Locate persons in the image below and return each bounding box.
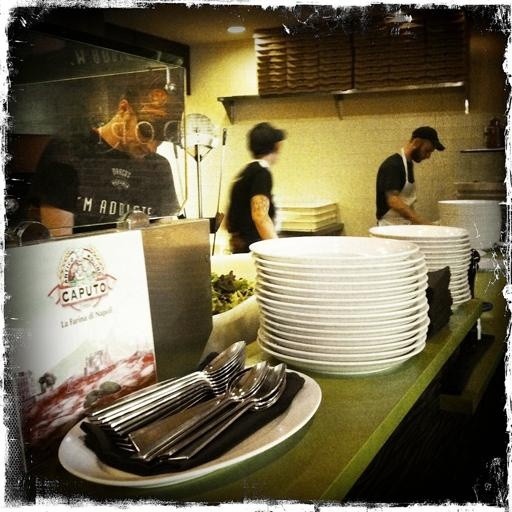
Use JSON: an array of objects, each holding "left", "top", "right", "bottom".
[
  {"left": 376, "top": 126, "right": 445, "bottom": 226},
  {"left": 227, "top": 122, "right": 288, "bottom": 253},
  {"left": 30, "top": 71, "right": 184, "bottom": 240}
]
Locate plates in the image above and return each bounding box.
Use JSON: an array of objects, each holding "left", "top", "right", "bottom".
[
  {"left": 61, "top": 366, "right": 331, "bottom": 487},
  {"left": 438, "top": 198, "right": 502, "bottom": 253},
  {"left": 369, "top": 223, "right": 476, "bottom": 314},
  {"left": 247, "top": 230, "right": 434, "bottom": 379}
]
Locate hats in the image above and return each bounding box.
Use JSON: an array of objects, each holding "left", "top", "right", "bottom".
[
  {"left": 250, "top": 122, "right": 284, "bottom": 152},
  {"left": 125, "top": 67, "right": 184, "bottom": 142},
  {"left": 411, "top": 126, "right": 446, "bottom": 151}
]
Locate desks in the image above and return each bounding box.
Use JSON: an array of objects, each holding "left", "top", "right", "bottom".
[{"left": 144, "top": 298, "right": 491, "bottom": 504}]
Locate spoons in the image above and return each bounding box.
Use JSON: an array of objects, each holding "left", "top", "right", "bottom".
[{"left": 82, "top": 342, "right": 288, "bottom": 471}]
